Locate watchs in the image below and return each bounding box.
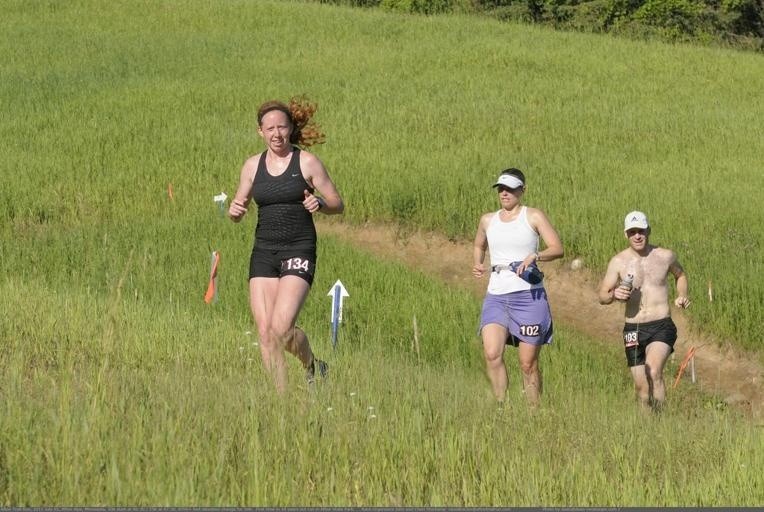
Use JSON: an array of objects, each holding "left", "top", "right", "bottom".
[{"left": 317, "top": 196, "right": 324, "bottom": 211}]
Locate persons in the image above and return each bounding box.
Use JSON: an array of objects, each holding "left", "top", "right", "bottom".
[
  {"left": 599, "top": 211, "right": 693, "bottom": 406},
  {"left": 472, "top": 168, "right": 564, "bottom": 402},
  {"left": 227, "top": 98, "right": 345, "bottom": 393}
]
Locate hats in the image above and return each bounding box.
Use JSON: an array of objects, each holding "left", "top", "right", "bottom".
[
  {"left": 492, "top": 174, "right": 524, "bottom": 190},
  {"left": 624, "top": 211, "right": 649, "bottom": 232}
]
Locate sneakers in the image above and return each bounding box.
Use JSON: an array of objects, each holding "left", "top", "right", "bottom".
[{"left": 307, "top": 359, "right": 329, "bottom": 387}]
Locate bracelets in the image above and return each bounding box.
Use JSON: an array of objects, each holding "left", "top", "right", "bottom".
[{"left": 535, "top": 252, "right": 539, "bottom": 260}]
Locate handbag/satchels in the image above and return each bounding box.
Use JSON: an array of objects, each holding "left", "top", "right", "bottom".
[{"left": 510, "top": 261, "right": 544, "bottom": 285}]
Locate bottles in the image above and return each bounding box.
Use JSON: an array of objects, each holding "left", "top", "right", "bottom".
[{"left": 619, "top": 274, "right": 633, "bottom": 303}]
